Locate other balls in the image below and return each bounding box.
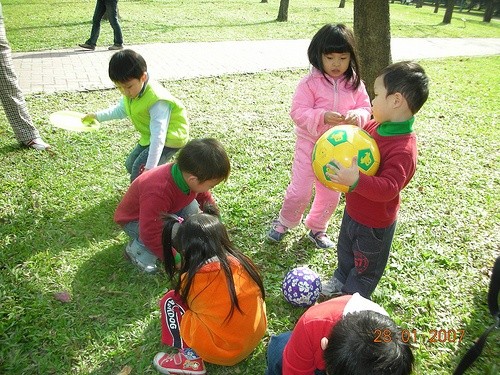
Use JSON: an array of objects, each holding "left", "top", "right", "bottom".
[
  {"left": 313, "top": 124, "right": 376, "bottom": 190},
  {"left": 284, "top": 266, "right": 320, "bottom": 305}
]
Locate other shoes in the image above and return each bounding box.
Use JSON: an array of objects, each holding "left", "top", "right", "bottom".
[
  {"left": 109, "top": 44, "right": 123, "bottom": 50},
  {"left": 309, "top": 229, "right": 334, "bottom": 249},
  {"left": 23, "top": 136, "right": 52, "bottom": 150},
  {"left": 78, "top": 40, "right": 96, "bottom": 50},
  {"left": 321, "top": 277, "right": 345, "bottom": 298},
  {"left": 124, "top": 244, "right": 159, "bottom": 274},
  {"left": 268, "top": 219, "right": 288, "bottom": 242},
  {"left": 153, "top": 347, "right": 208, "bottom": 375}
]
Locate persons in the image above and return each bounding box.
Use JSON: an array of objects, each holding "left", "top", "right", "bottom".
[
  {"left": 81, "top": 49, "right": 189, "bottom": 183},
  {"left": 152, "top": 197, "right": 269, "bottom": 375},
  {"left": 263, "top": 291, "right": 417, "bottom": 375},
  {"left": 114, "top": 138, "right": 231, "bottom": 272},
  {"left": 318, "top": 60, "right": 429, "bottom": 300},
  {"left": 266, "top": 23, "right": 374, "bottom": 249},
  {"left": 0, "top": 2, "right": 52, "bottom": 150},
  {"left": 78, "top": 0, "right": 124, "bottom": 50}
]
